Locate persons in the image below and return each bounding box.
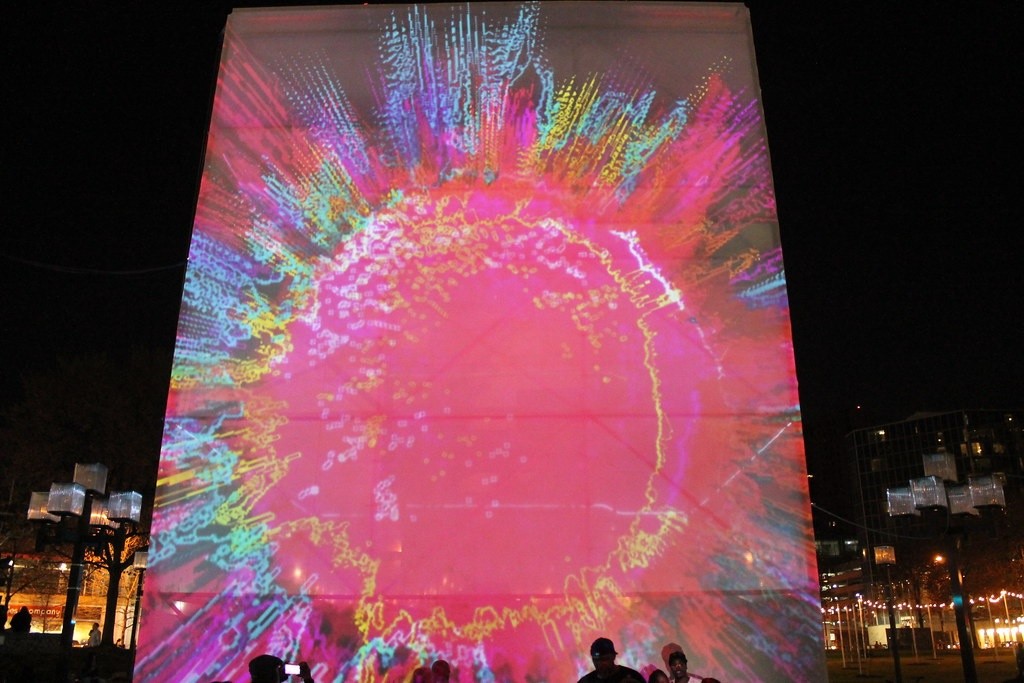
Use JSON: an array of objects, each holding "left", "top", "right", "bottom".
[
  {"left": 249, "top": 655, "right": 314, "bottom": 683},
  {"left": 649, "top": 651, "right": 720, "bottom": 683},
  {"left": 88, "top": 623, "right": 101, "bottom": 646},
  {"left": 577, "top": 638, "right": 646, "bottom": 683},
  {"left": 410, "top": 660, "right": 450, "bottom": 683},
  {"left": 1016, "top": 643, "right": 1024, "bottom": 675},
  {"left": 0, "top": 596, "right": 32, "bottom": 634}
]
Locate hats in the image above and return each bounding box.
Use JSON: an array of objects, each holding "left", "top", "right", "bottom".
[{"left": 590, "top": 637, "right": 618, "bottom": 656}]
